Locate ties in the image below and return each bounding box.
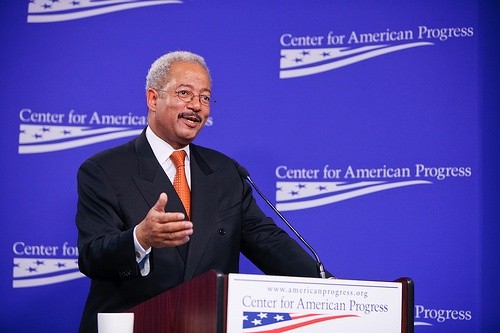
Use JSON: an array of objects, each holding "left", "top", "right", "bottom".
[{"left": 169, "top": 150, "right": 191, "bottom": 221}]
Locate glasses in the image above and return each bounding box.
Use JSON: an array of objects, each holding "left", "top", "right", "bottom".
[{"left": 157, "top": 90, "right": 216, "bottom": 107}]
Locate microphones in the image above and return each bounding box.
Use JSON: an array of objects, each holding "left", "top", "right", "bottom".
[{"left": 237, "top": 165, "right": 326, "bottom": 279}]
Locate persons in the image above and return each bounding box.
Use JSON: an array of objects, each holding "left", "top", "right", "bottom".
[{"left": 75, "top": 51, "right": 336, "bottom": 333}]
